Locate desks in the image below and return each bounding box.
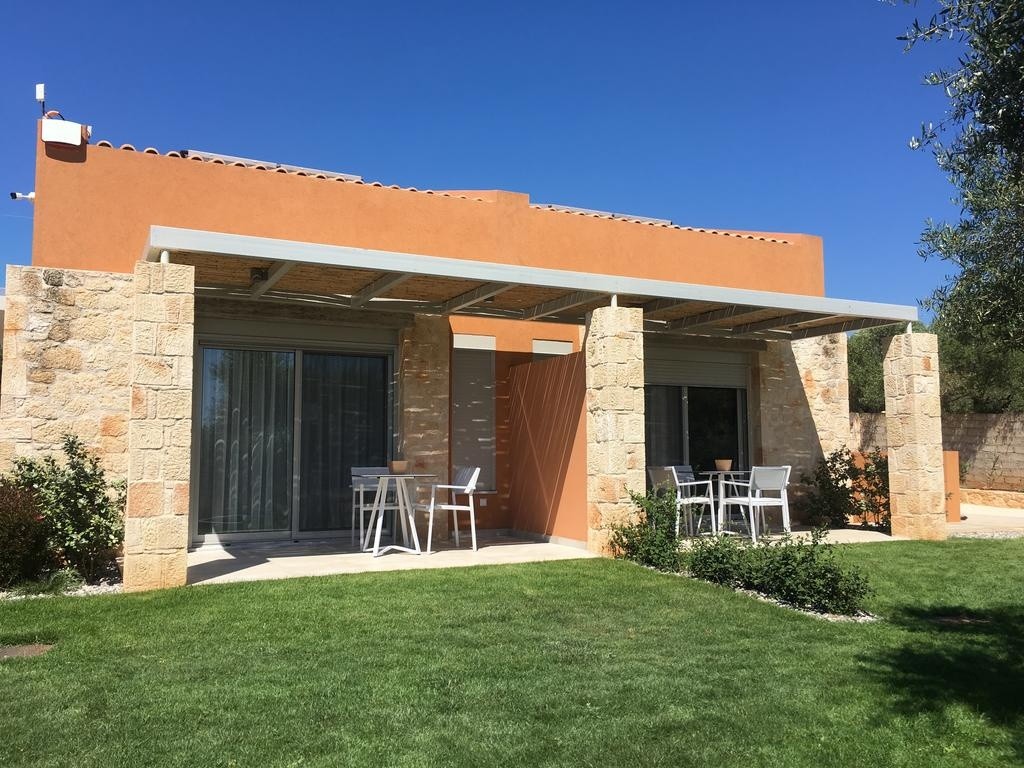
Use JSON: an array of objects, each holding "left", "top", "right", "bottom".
[
  {"left": 352, "top": 473, "right": 437, "bottom": 557},
  {"left": 677, "top": 469, "right": 766, "bottom": 540}
]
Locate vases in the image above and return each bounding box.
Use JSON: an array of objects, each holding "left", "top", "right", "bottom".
[
  {"left": 389, "top": 460, "right": 410, "bottom": 474},
  {"left": 715, "top": 459, "right": 732, "bottom": 470}
]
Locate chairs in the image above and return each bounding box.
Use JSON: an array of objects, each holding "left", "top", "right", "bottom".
[
  {"left": 349, "top": 465, "right": 415, "bottom": 551},
  {"left": 411, "top": 466, "right": 482, "bottom": 553},
  {"left": 647, "top": 465, "right": 792, "bottom": 538}
]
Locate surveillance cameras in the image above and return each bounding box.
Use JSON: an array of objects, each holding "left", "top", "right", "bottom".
[{"left": 10, "top": 192, "right": 23, "bottom": 200}]
